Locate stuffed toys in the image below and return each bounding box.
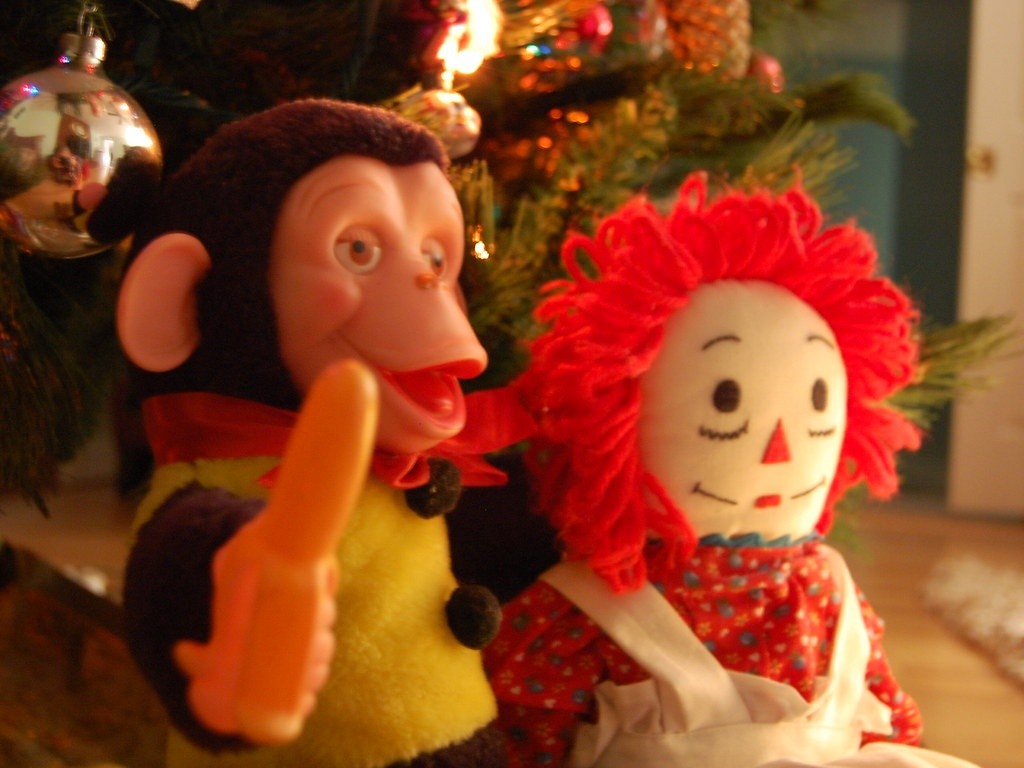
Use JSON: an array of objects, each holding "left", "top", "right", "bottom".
[
  {"left": 117, "top": 100, "right": 510, "bottom": 768},
  {"left": 464, "top": 170, "right": 925, "bottom": 768}
]
